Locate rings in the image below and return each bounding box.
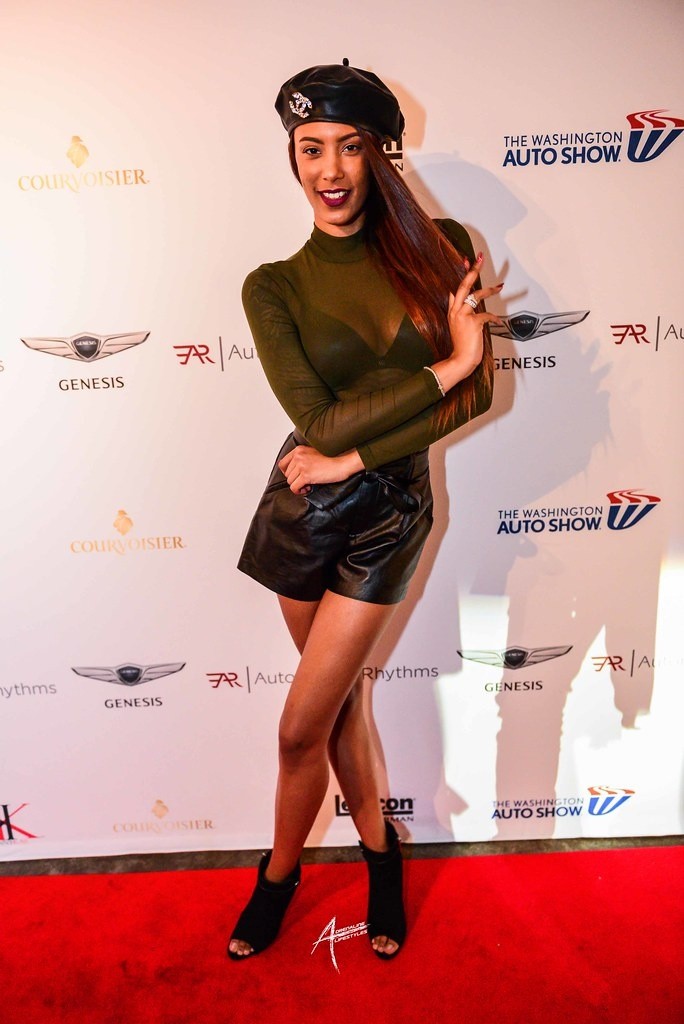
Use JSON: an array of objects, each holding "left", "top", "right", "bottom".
[{"left": 463, "top": 293, "right": 479, "bottom": 308}]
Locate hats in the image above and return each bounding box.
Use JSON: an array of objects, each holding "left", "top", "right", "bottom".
[{"left": 273, "top": 59, "right": 404, "bottom": 137}]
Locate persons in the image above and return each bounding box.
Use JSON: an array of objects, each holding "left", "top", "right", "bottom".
[{"left": 228, "top": 57, "right": 505, "bottom": 959}]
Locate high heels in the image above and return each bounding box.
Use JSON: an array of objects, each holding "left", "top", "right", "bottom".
[
  {"left": 226, "top": 850, "right": 304, "bottom": 961},
  {"left": 356, "top": 823, "right": 407, "bottom": 958}
]
